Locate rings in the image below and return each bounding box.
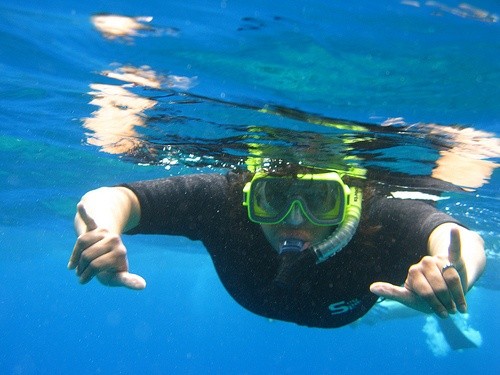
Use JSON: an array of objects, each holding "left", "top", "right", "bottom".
[{"left": 441, "top": 264, "right": 457, "bottom": 275}]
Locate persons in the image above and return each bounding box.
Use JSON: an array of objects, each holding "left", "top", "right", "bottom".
[{"left": 64, "top": 161, "right": 487, "bottom": 351}]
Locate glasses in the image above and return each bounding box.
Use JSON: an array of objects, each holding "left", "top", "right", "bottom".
[{"left": 243, "top": 170, "right": 351, "bottom": 226}]
